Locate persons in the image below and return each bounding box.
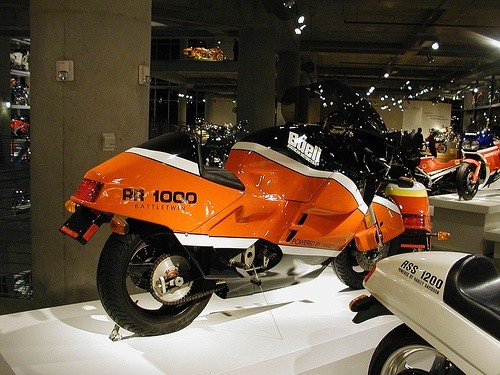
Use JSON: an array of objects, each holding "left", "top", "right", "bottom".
[
  {"left": 401, "top": 131, "right": 409, "bottom": 146},
  {"left": 413, "top": 127, "right": 423, "bottom": 151},
  {"left": 428, "top": 132, "right": 438, "bottom": 158},
  {"left": 408, "top": 128, "right": 417, "bottom": 140}
]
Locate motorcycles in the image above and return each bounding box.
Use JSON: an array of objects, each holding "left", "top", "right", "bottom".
[
  {"left": 183, "top": 117, "right": 500, "bottom": 253},
  {"left": 349, "top": 251, "right": 500, "bottom": 374},
  {"left": 58, "top": 59, "right": 451, "bottom": 336}
]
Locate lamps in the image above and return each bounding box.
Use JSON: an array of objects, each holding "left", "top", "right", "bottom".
[{"left": 432, "top": 35, "right": 440, "bottom": 50}]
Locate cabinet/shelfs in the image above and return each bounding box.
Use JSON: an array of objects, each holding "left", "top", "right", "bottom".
[
  {"left": 430, "top": 71, "right": 500, "bottom": 138},
  {"left": 0, "top": 0, "right": 33, "bottom": 301}
]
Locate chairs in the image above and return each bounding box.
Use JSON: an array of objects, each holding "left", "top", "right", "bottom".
[
  {"left": 322, "top": 154, "right": 387, "bottom": 181},
  {"left": 442, "top": 254, "right": 500, "bottom": 342},
  {"left": 196, "top": 135, "right": 245, "bottom": 189}
]
